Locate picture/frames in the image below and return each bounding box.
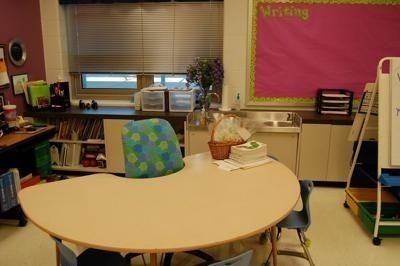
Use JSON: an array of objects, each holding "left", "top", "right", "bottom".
[{"left": 12, "top": 72, "right": 30, "bottom": 96}]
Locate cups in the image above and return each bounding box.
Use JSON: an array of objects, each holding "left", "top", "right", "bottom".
[{"left": 2, "top": 104, "right": 17, "bottom": 122}]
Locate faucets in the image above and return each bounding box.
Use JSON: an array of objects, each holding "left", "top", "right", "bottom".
[{"left": 205, "top": 93, "right": 220, "bottom": 103}]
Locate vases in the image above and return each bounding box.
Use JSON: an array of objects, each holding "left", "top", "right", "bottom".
[{"left": 200, "top": 94, "right": 212, "bottom": 112}]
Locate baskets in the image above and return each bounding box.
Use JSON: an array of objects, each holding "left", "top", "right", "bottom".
[{"left": 208, "top": 114, "right": 247, "bottom": 160}]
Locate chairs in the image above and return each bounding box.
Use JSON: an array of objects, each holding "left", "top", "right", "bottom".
[
  {"left": 121, "top": 118, "right": 214, "bottom": 266},
  {"left": 194, "top": 249, "right": 253, "bottom": 265},
  {"left": 265, "top": 179, "right": 315, "bottom": 266},
  {"left": 49, "top": 234, "right": 124, "bottom": 266}
]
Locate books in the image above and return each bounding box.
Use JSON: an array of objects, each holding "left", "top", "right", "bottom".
[
  {"left": 24, "top": 80, "right": 50, "bottom": 108},
  {"left": 33, "top": 115, "right": 104, "bottom": 167},
  {"left": 223, "top": 140, "right": 275, "bottom": 170}
]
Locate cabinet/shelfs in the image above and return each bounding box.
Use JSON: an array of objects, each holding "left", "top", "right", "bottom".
[
  {"left": 22, "top": 106, "right": 197, "bottom": 177},
  {"left": 298, "top": 122, "right": 357, "bottom": 187},
  {"left": 184, "top": 132, "right": 302, "bottom": 176},
  {"left": 316, "top": 88, "right": 354, "bottom": 116}
]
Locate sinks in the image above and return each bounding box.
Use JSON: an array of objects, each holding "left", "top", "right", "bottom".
[{"left": 264, "top": 121, "right": 303, "bottom": 133}]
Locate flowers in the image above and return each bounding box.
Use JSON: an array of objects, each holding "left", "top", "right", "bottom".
[{"left": 185, "top": 56, "right": 223, "bottom": 96}]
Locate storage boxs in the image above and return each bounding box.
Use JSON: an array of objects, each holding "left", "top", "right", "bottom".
[
  {"left": 358, "top": 203, "right": 400, "bottom": 235},
  {"left": 345, "top": 188, "right": 397, "bottom": 216}
]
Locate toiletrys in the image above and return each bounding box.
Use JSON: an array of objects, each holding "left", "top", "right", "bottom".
[{"left": 235, "top": 93, "right": 240, "bottom": 111}]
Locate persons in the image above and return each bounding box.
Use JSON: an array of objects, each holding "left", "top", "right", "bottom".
[{"left": 51, "top": 86, "right": 68, "bottom": 107}]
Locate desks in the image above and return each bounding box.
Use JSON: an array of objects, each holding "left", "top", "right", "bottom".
[
  {"left": 17, "top": 152, "right": 301, "bottom": 266},
  {"left": 0, "top": 124, "right": 57, "bottom": 226}
]
